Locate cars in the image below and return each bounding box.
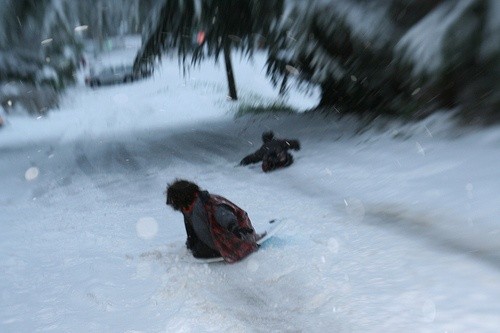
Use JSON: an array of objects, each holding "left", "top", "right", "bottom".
[{"left": 84, "top": 62, "right": 151, "bottom": 89}]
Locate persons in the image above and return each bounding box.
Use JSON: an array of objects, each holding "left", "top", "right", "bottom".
[
  {"left": 239, "top": 129, "right": 300, "bottom": 172},
  {"left": 167, "top": 180, "right": 267, "bottom": 263}
]
